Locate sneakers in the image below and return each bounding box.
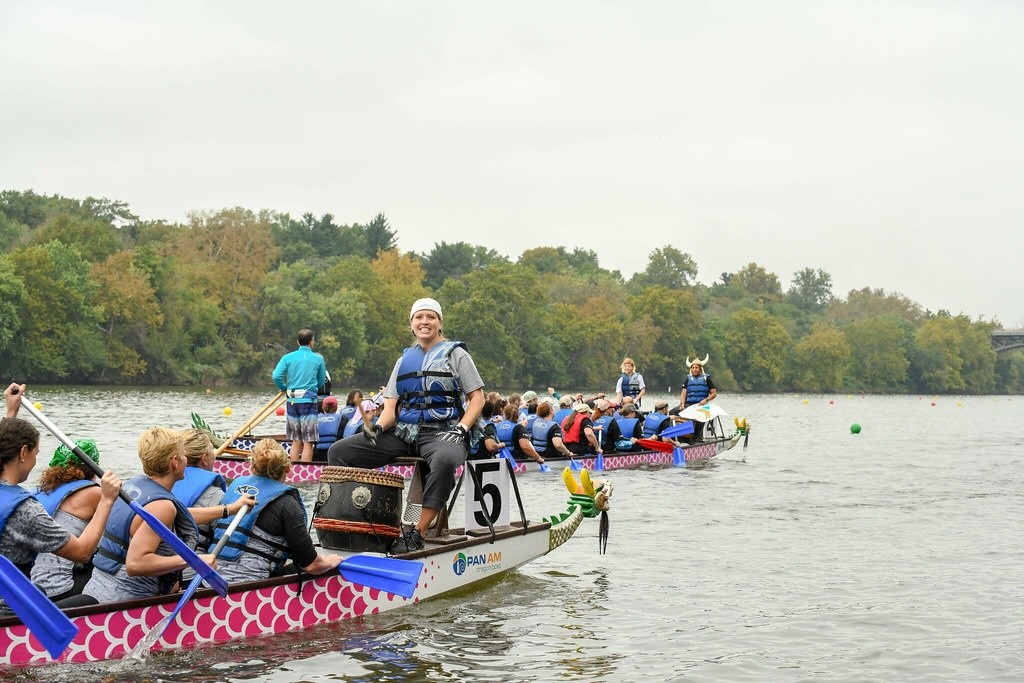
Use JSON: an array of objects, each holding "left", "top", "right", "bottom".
[{"left": 391, "top": 523, "right": 425, "bottom": 555}]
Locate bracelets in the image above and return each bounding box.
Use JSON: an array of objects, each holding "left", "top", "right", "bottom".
[
  {"left": 457, "top": 422, "right": 469, "bottom": 433},
  {"left": 706, "top": 398, "right": 708, "bottom": 402},
  {"left": 595, "top": 395, "right": 598, "bottom": 399},
  {"left": 221, "top": 504, "right": 228, "bottom": 518}
]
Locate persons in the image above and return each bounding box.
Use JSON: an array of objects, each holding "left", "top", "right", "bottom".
[
  {"left": 615, "top": 358, "right": 646, "bottom": 407},
  {"left": 0, "top": 382, "right": 343, "bottom": 614},
  {"left": 271, "top": 329, "right": 325, "bottom": 462},
  {"left": 327, "top": 298, "right": 485, "bottom": 554},
  {"left": 314, "top": 387, "right": 694, "bottom": 463},
  {"left": 669, "top": 359, "right": 717, "bottom": 445}
]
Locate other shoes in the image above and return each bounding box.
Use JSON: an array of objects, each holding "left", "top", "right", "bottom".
[{"left": 688, "top": 438, "right": 696, "bottom": 445}]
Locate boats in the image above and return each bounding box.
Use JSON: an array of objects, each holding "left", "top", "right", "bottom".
[
  {"left": 211, "top": 414, "right": 752, "bottom": 483},
  {"left": 0, "top": 464, "right": 615, "bottom": 669}
]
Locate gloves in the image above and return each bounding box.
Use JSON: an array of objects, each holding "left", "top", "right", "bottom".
[
  {"left": 434, "top": 422, "right": 469, "bottom": 444},
  {"left": 363, "top": 422, "right": 383, "bottom": 446}
]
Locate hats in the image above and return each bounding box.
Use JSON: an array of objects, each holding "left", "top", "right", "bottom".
[
  {"left": 687, "top": 353, "right": 709, "bottom": 381},
  {"left": 522, "top": 390, "right": 537, "bottom": 404},
  {"left": 410, "top": 298, "right": 443, "bottom": 320},
  {"left": 372, "top": 395, "right": 384, "bottom": 406},
  {"left": 622, "top": 402, "right": 636, "bottom": 413},
  {"left": 323, "top": 396, "right": 337, "bottom": 408},
  {"left": 622, "top": 396, "right": 635, "bottom": 404},
  {"left": 655, "top": 402, "right": 667, "bottom": 410},
  {"left": 352, "top": 401, "right": 379, "bottom": 424},
  {"left": 573, "top": 404, "right": 595, "bottom": 414},
  {"left": 560, "top": 395, "right": 573, "bottom": 407},
  {"left": 607, "top": 399, "right": 616, "bottom": 408},
  {"left": 597, "top": 401, "right": 610, "bottom": 411}
]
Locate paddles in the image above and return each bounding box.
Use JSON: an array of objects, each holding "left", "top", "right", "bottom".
[
  {"left": 673, "top": 417, "right": 686, "bottom": 464},
  {"left": 11, "top": 386, "right": 229, "bottom": 598},
  {"left": 657, "top": 420, "right": 695, "bottom": 437},
  {"left": 338, "top": 554, "right": 425, "bottom": 599},
  {"left": 216, "top": 392, "right": 288, "bottom": 457},
  {"left": 495, "top": 436, "right": 517, "bottom": 469},
  {"left": 0, "top": 555, "right": 80, "bottom": 661},
  {"left": 146, "top": 505, "right": 248, "bottom": 645},
  {"left": 538, "top": 462, "right": 550, "bottom": 472},
  {"left": 569, "top": 456, "right": 583, "bottom": 470},
  {"left": 622, "top": 436, "right": 674, "bottom": 455},
  {"left": 594, "top": 429, "right": 604, "bottom": 470}
]
